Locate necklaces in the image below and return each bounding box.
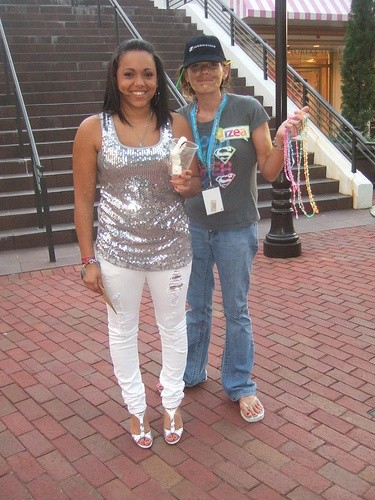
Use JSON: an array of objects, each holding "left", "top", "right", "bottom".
[
  {"left": 281, "top": 111, "right": 319, "bottom": 219},
  {"left": 121, "top": 110, "right": 155, "bottom": 149}
]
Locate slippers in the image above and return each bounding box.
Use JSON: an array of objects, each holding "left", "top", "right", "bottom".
[{"left": 239, "top": 395, "right": 264, "bottom": 422}]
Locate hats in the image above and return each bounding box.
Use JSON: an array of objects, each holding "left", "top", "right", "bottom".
[{"left": 182, "top": 35, "right": 224, "bottom": 67}]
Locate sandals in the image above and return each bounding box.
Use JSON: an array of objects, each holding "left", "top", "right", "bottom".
[
  {"left": 162, "top": 407, "right": 184, "bottom": 444},
  {"left": 130, "top": 411, "right": 153, "bottom": 449}
]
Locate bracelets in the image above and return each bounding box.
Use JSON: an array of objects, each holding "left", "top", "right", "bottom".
[{"left": 81, "top": 257, "right": 96, "bottom": 267}]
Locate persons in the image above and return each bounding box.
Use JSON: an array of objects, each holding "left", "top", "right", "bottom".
[
  {"left": 71, "top": 38, "right": 203, "bottom": 448},
  {"left": 176, "top": 34, "right": 310, "bottom": 422}
]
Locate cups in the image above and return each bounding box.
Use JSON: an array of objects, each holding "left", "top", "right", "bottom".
[{"left": 167, "top": 138, "right": 199, "bottom": 186}]
